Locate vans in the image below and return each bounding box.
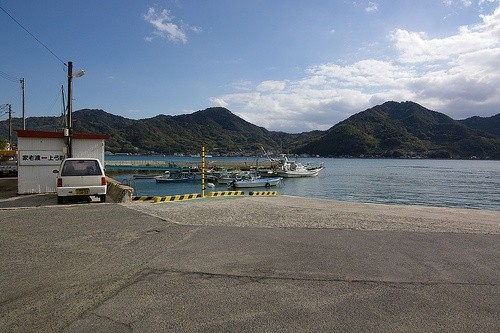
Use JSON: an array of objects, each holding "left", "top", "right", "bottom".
[{"left": 52, "top": 158, "right": 108, "bottom": 205}]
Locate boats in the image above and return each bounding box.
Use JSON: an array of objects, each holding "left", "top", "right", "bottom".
[
  {"left": 233, "top": 172, "right": 284, "bottom": 187},
  {"left": 260, "top": 145, "right": 326, "bottom": 177},
  {"left": 132, "top": 162, "right": 273, "bottom": 185}
]
